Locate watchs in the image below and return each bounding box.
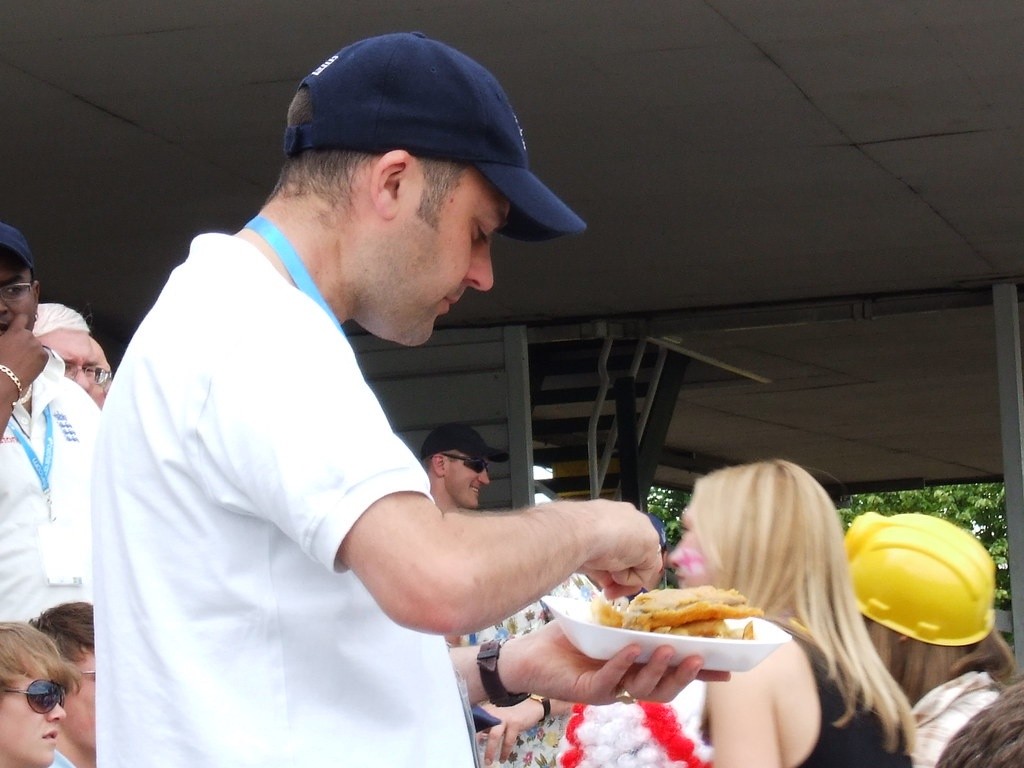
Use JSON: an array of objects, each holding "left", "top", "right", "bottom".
[
  {"left": 477, "top": 639, "right": 532, "bottom": 707},
  {"left": 530, "top": 693, "right": 551, "bottom": 723}
]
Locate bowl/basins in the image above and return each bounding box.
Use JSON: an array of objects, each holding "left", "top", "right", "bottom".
[{"left": 539, "top": 596, "right": 792, "bottom": 672}]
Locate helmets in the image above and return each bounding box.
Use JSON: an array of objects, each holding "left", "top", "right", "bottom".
[{"left": 843, "top": 511, "right": 998, "bottom": 647}]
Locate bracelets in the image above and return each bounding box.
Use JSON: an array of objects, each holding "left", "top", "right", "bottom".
[{"left": 0, "top": 365, "right": 22, "bottom": 400}]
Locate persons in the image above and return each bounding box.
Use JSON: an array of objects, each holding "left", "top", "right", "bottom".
[
  {"left": 839, "top": 511, "right": 1024, "bottom": 768},
  {"left": 92, "top": 31, "right": 662, "bottom": 768},
  {"left": 669, "top": 460, "right": 917, "bottom": 768},
  {"left": 0, "top": 224, "right": 112, "bottom": 768}
]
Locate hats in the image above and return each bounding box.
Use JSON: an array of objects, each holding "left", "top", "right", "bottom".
[
  {"left": 420, "top": 422, "right": 509, "bottom": 464},
  {"left": 283, "top": 30, "right": 589, "bottom": 244},
  {"left": 639, "top": 510, "right": 666, "bottom": 548},
  {"left": 0, "top": 222, "right": 34, "bottom": 280}
]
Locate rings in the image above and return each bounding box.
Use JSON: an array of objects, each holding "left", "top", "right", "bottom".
[{"left": 657, "top": 544, "right": 662, "bottom": 553}]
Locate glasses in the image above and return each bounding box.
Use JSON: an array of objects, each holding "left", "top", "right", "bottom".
[
  {"left": 1, "top": 678, "right": 65, "bottom": 714},
  {"left": 0, "top": 282, "right": 33, "bottom": 302},
  {"left": 64, "top": 363, "right": 100, "bottom": 384},
  {"left": 430, "top": 453, "right": 489, "bottom": 473},
  {"left": 95, "top": 367, "right": 112, "bottom": 384}
]
